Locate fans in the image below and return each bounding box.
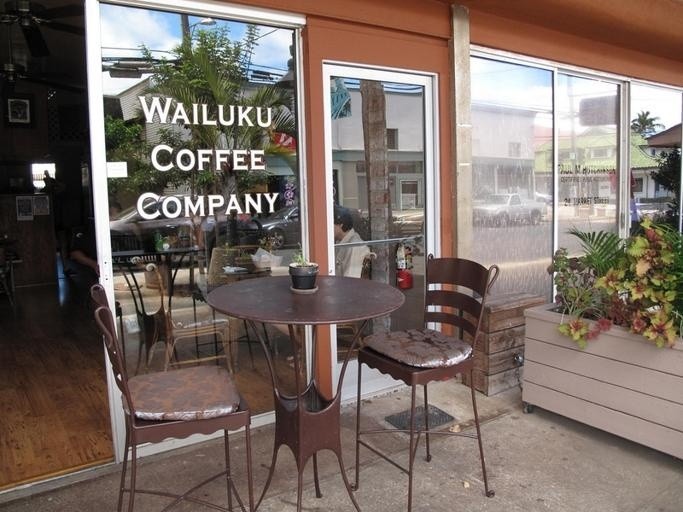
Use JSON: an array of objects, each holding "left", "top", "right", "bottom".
[
  {"left": 0, "top": 0, "right": 85, "bottom": 58},
  {"left": 0, "top": 63, "right": 87, "bottom": 93}
]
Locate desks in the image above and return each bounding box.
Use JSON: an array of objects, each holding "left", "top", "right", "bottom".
[
  {"left": 109, "top": 246, "right": 205, "bottom": 377},
  {"left": 205, "top": 275, "right": 406, "bottom": 512}
]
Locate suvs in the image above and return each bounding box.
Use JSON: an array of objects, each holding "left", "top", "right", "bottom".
[{"left": 109, "top": 193, "right": 265, "bottom": 266}]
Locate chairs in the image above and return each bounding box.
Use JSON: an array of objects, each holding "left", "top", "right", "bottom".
[
  {"left": 347, "top": 253, "right": 501, "bottom": 512},
  {"left": 61, "top": 217, "right": 97, "bottom": 308},
  {"left": 90, "top": 284, "right": 253, "bottom": 512},
  {"left": 115, "top": 257, "right": 233, "bottom": 380}
]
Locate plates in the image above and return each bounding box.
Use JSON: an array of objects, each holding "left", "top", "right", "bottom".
[{"left": 289, "top": 285, "right": 320, "bottom": 296}]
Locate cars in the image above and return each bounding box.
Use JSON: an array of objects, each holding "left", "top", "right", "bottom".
[
  {"left": 393, "top": 213, "right": 426, "bottom": 235},
  {"left": 248, "top": 203, "right": 362, "bottom": 250}
]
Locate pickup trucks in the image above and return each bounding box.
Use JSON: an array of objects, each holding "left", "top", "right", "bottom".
[{"left": 474, "top": 193, "right": 547, "bottom": 227}]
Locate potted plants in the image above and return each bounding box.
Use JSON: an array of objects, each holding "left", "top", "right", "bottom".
[{"left": 288, "top": 248, "right": 319, "bottom": 294}]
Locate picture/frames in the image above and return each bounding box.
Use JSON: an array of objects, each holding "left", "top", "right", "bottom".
[{"left": 2, "top": 91, "right": 36, "bottom": 129}]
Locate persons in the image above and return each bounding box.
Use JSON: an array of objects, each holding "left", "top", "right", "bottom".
[
  {"left": 65, "top": 204, "right": 142, "bottom": 328},
  {"left": 335, "top": 205, "right": 366, "bottom": 279}
]
